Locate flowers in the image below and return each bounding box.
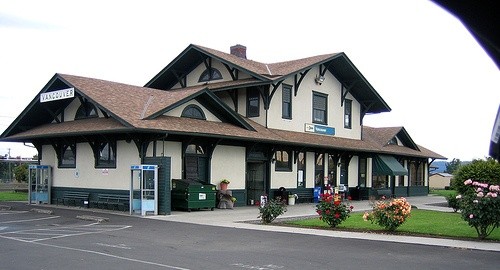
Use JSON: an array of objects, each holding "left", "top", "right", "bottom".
[
  {"left": 318, "top": 194, "right": 354, "bottom": 226},
  {"left": 458, "top": 182, "right": 500, "bottom": 238},
  {"left": 363, "top": 197, "right": 412, "bottom": 230},
  {"left": 256, "top": 197, "right": 287, "bottom": 222}
]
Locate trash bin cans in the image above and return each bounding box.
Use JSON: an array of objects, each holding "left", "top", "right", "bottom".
[{"left": 171, "top": 177, "right": 217, "bottom": 212}]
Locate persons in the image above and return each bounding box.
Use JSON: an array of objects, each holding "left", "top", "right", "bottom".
[{"left": 278, "top": 187, "right": 287, "bottom": 204}]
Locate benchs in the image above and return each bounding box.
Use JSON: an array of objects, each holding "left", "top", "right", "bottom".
[
  {"left": 95, "top": 193, "right": 130, "bottom": 211},
  {"left": 62, "top": 191, "right": 89, "bottom": 208}
]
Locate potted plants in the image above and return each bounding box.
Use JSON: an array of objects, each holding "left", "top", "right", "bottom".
[{"left": 220, "top": 179, "right": 230, "bottom": 190}]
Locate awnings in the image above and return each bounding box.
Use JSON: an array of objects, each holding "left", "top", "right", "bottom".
[{"left": 372, "top": 156, "right": 408, "bottom": 177}]
